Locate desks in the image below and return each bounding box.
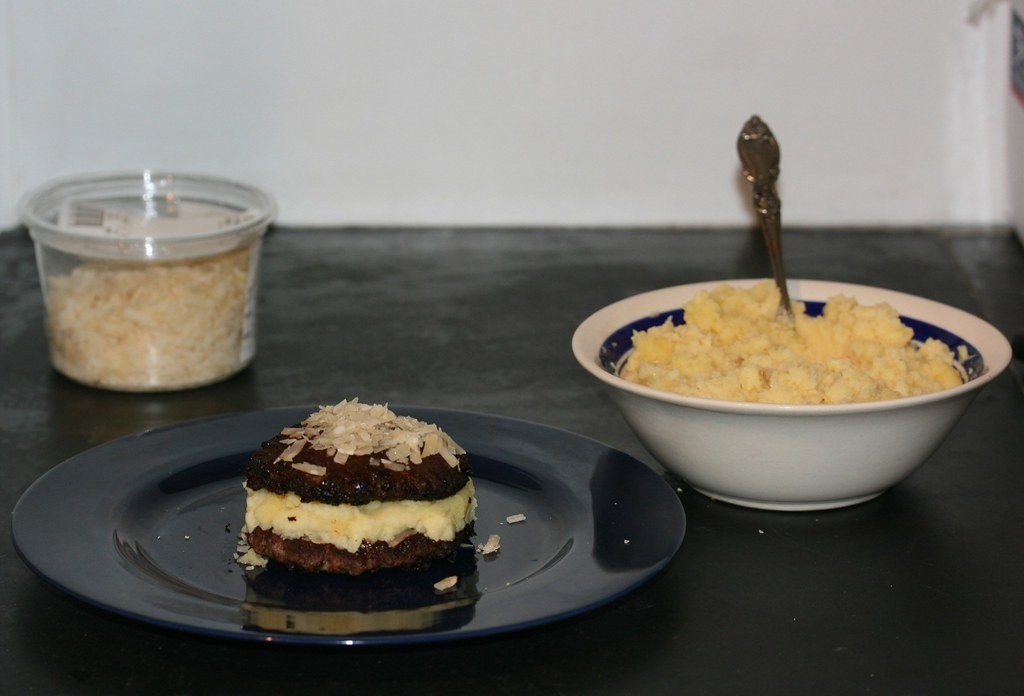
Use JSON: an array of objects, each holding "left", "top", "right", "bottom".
[{"left": 0, "top": 213, "right": 1024, "bottom": 696}]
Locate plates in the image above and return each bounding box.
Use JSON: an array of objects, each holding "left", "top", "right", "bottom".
[{"left": 13, "top": 406, "right": 685, "bottom": 645}]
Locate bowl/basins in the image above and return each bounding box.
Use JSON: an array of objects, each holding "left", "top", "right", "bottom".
[
  {"left": 572, "top": 278, "right": 1011, "bottom": 510},
  {"left": 25, "top": 173, "right": 277, "bottom": 391}
]
